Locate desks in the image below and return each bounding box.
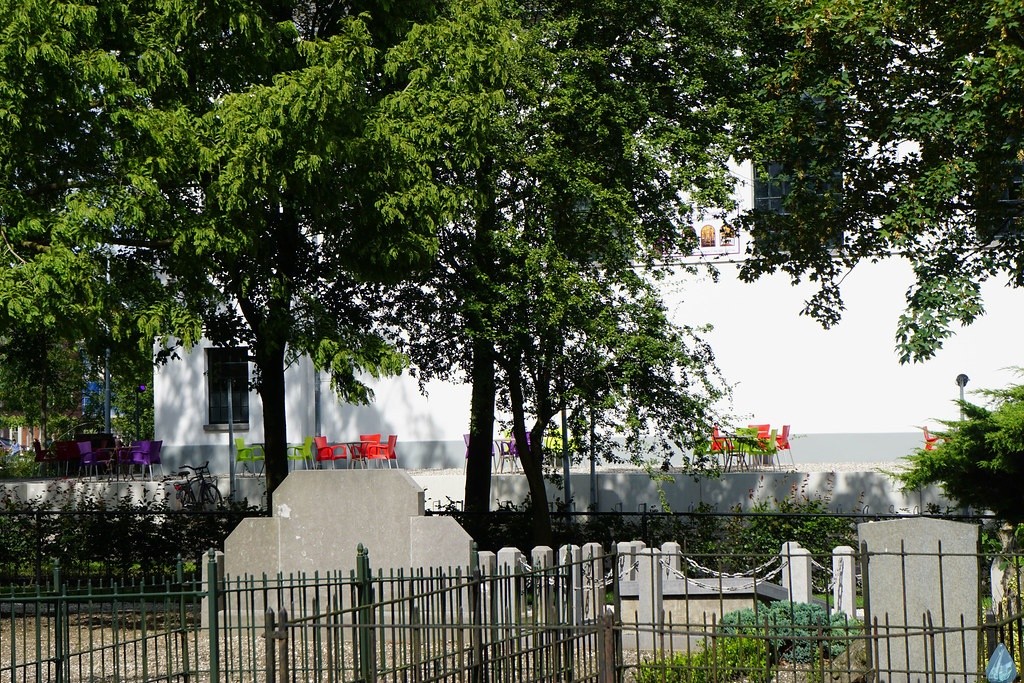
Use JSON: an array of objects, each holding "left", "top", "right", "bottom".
[
  {"left": 251, "top": 442, "right": 290, "bottom": 477},
  {"left": 338, "top": 441, "right": 376, "bottom": 469},
  {"left": 99, "top": 446, "right": 140, "bottom": 481},
  {"left": 714, "top": 436, "right": 753, "bottom": 471},
  {"left": 493, "top": 439, "right": 520, "bottom": 473}
]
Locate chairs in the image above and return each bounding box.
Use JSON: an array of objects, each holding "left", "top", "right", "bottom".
[
  {"left": 233, "top": 432, "right": 399, "bottom": 476},
  {"left": 924, "top": 425, "right": 949, "bottom": 453},
  {"left": 31, "top": 436, "right": 163, "bottom": 482},
  {"left": 687, "top": 423, "right": 796, "bottom": 471},
  {"left": 465, "top": 426, "right": 585, "bottom": 473}
]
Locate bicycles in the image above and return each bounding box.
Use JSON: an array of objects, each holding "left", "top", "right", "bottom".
[{"left": 172, "top": 460, "right": 225, "bottom": 511}]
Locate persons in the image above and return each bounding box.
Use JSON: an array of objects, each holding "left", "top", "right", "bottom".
[
  {"left": 9, "top": 439, "right": 20, "bottom": 456},
  {"left": 32, "top": 438, "right": 41, "bottom": 452}
]
